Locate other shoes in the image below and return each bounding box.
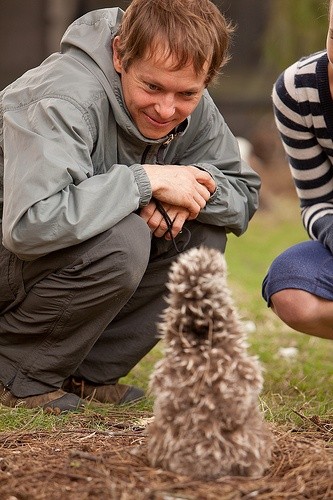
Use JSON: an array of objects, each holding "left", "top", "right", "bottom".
[
  {"left": 0, "top": 388, "right": 83, "bottom": 416},
  {"left": 67, "top": 381, "right": 147, "bottom": 409}
]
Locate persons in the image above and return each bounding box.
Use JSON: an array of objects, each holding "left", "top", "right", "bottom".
[
  {"left": 1, "top": 0, "right": 265, "bottom": 413},
  {"left": 260, "top": 1, "right": 332, "bottom": 340}
]
